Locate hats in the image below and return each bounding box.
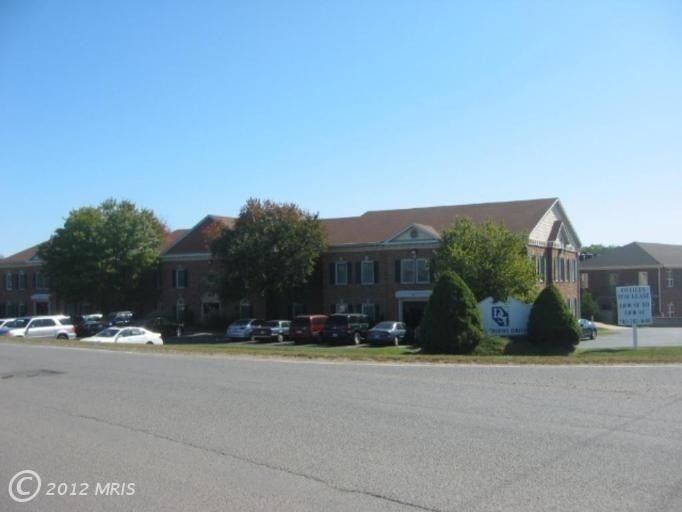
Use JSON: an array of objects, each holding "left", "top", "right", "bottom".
[
  {"left": 227, "top": 313, "right": 410, "bottom": 347},
  {"left": 574, "top": 318, "right": 597, "bottom": 340},
  {"left": 0, "top": 310, "right": 182, "bottom": 344}
]
